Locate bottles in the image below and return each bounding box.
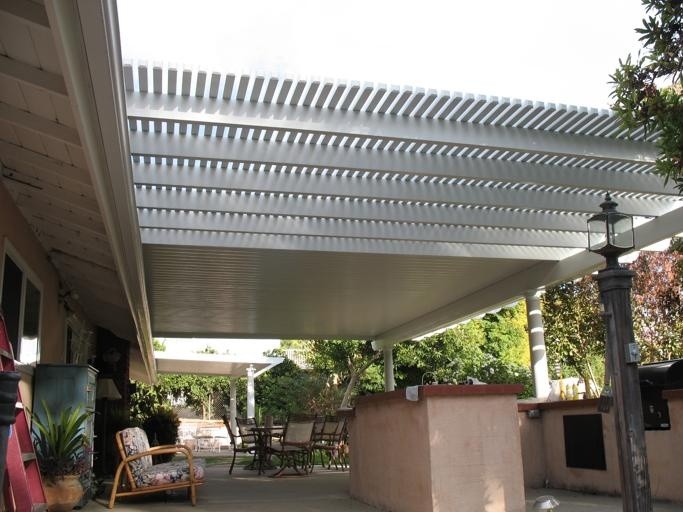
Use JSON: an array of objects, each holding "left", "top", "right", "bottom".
[{"left": 559, "top": 379, "right": 579, "bottom": 401}]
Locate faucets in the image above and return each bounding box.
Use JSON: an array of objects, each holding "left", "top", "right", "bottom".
[{"left": 422, "top": 372, "right": 436, "bottom": 385}]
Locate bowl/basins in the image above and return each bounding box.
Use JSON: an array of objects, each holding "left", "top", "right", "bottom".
[{"left": 532, "top": 495, "right": 560, "bottom": 512}]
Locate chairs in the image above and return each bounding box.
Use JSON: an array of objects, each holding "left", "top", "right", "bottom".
[
  {"left": 220, "top": 409, "right": 354, "bottom": 478},
  {"left": 108, "top": 423, "right": 205, "bottom": 510}
]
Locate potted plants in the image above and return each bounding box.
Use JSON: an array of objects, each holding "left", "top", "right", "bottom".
[{"left": 22, "top": 398, "right": 98, "bottom": 512}]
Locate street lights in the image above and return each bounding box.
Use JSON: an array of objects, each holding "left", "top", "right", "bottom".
[{"left": 586, "top": 192, "right": 654, "bottom": 512}]
